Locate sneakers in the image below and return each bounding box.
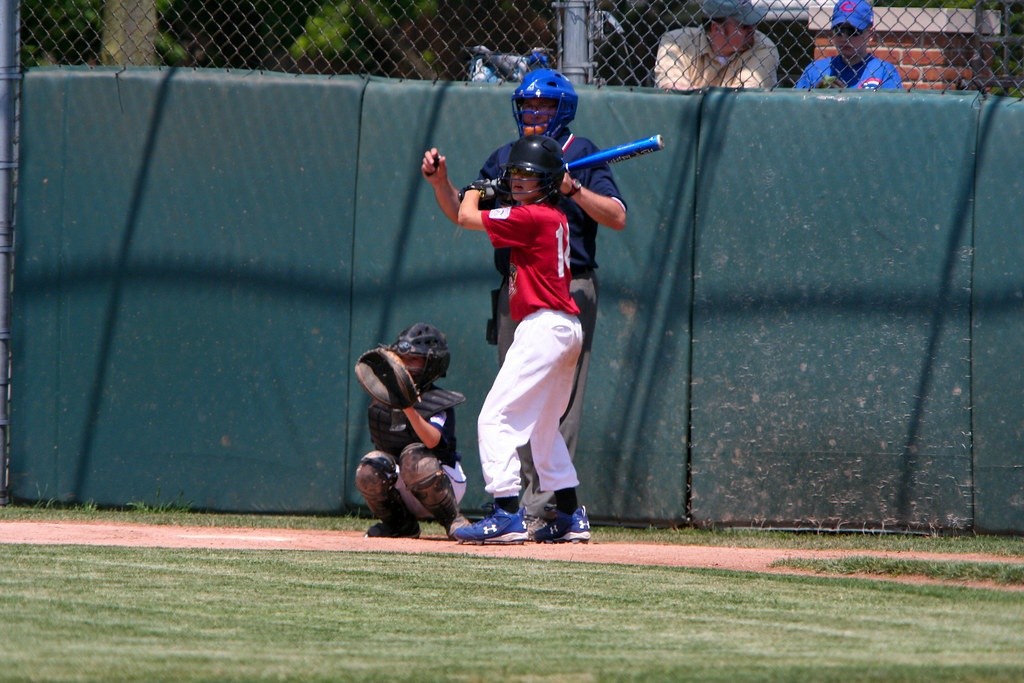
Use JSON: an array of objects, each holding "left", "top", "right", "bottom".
[
  {"left": 534, "top": 503, "right": 591, "bottom": 543},
  {"left": 453, "top": 502, "right": 528, "bottom": 545}
]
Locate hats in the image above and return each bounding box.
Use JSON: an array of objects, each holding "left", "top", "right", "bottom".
[
  {"left": 702, "top": 0, "right": 766, "bottom": 26},
  {"left": 830, "top": 0, "right": 874, "bottom": 30}
]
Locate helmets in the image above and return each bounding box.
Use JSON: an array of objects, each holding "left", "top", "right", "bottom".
[
  {"left": 512, "top": 67, "right": 577, "bottom": 138},
  {"left": 393, "top": 323, "right": 449, "bottom": 387},
  {"left": 502, "top": 134, "right": 566, "bottom": 190}
]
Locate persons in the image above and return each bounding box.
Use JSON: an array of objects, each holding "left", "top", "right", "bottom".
[
  {"left": 353, "top": 323, "right": 473, "bottom": 540},
  {"left": 419, "top": 69, "right": 630, "bottom": 541},
  {"left": 796, "top": 0, "right": 904, "bottom": 90},
  {"left": 450, "top": 133, "right": 591, "bottom": 545},
  {"left": 653, "top": 0, "right": 781, "bottom": 89}
]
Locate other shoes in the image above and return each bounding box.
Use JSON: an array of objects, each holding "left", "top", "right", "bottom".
[
  {"left": 447, "top": 518, "right": 472, "bottom": 539},
  {"left": 369, "top": 522, "right": 421, "bottom": 539}
]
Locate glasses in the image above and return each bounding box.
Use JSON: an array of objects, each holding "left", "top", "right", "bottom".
[
  {"left": 507, "top": 165, "right": 545, "bottom": 178},
  {"left": 518, "top": 103, "right": 558, "bottom": 112},
  {"left": 834, "top": 25, "right": 862, "bottom": 36}
]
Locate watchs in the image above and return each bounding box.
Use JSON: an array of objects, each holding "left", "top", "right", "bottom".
[{"left": 565, "top": 178, "right": 581, "bottom": 199}]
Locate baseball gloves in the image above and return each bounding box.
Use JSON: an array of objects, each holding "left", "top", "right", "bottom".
[{"left": 353, "top": 346, "right": 418, "bottom": 410}]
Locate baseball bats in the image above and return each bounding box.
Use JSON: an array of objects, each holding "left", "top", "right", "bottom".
[{"left": 457, "top": 134, "right": 666, "bottom": 203}]
